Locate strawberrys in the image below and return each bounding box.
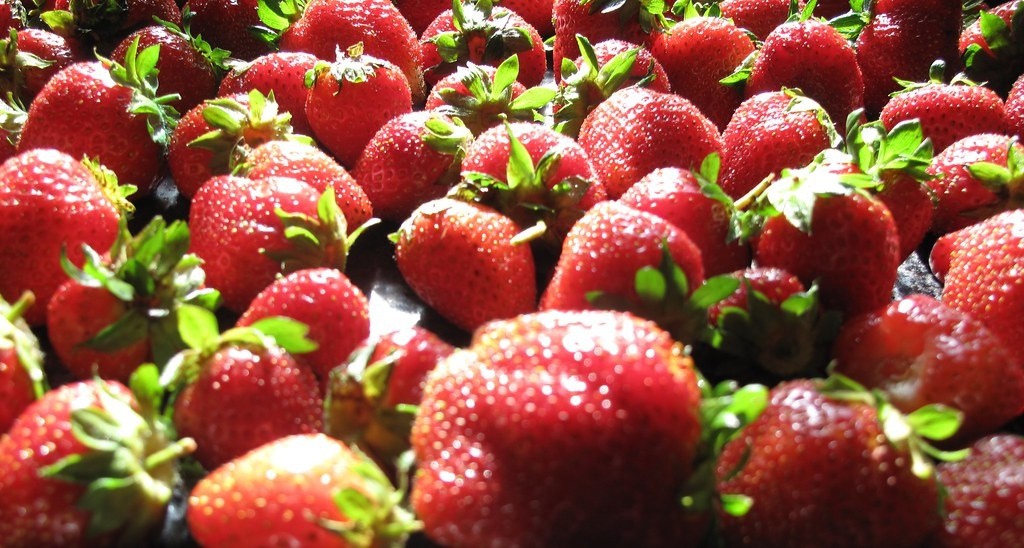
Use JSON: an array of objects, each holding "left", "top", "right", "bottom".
[{"left": 1, "top": 1, "right": 1024, "bottom": 546}]
